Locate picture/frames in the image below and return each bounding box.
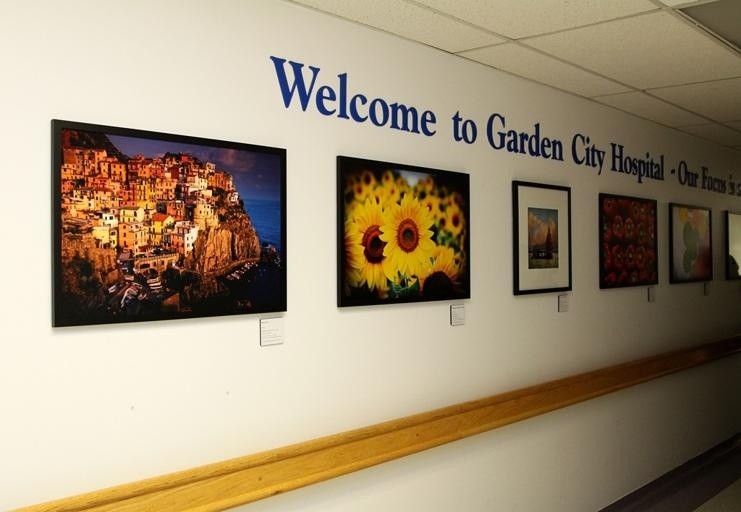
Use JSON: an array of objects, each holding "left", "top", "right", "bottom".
[
  {"left": 51, "top": 118, "right": 287, "bottom": 328},
  {"left": 336, "top": 154, "right": 470, "bottom": 309},
  {"left": 597, "top": 191, "right": 740, "bottom": 290},
  {"left": 512, "top": 179, "right": 572, "bottom": 294}
]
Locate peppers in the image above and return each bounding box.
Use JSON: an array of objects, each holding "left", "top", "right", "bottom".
[{"left": 604, "top": 197, "right": 659, "bottom": 286}]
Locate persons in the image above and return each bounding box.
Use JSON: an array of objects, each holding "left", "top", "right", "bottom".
[{"left": 682, "top": 209, "right": 702, "bottom": 273}]
